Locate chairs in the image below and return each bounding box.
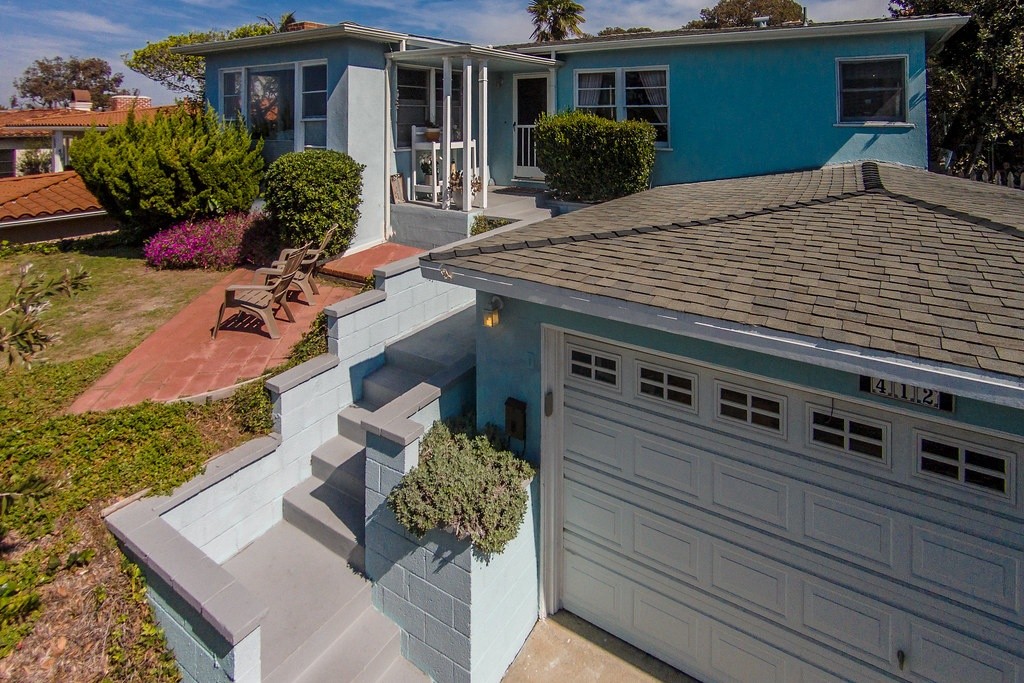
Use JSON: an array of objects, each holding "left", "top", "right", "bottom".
[
  {"left": 265, "top": 223, "right": 339, "bottom": 306},
  {"left": 211, "top": 240, "right": 314, "bottom": 340}
]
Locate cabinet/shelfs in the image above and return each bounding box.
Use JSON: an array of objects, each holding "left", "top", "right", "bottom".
[{"left": 411, "top": 126, "right": 477, "bottom": 203}]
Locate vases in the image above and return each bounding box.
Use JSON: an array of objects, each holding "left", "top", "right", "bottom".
[{"left": 423, "top": 175, "right": 433, "bottom": 185}]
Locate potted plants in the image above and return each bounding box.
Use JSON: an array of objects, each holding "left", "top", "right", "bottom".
[
  {"left": 425, "top": 118, "right": 440, "bottom": 141},
  {"left": 447, "top": 170, "right": 483, "bottom": 208}
]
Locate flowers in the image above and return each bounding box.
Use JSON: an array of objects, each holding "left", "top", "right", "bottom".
[{"left": 418, "top": 153, "right": 440, "bottom": 174}]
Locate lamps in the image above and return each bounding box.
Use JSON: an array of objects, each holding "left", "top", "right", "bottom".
[{"left": 483, "top": 296, "right": 504, "bottom": 327}]
